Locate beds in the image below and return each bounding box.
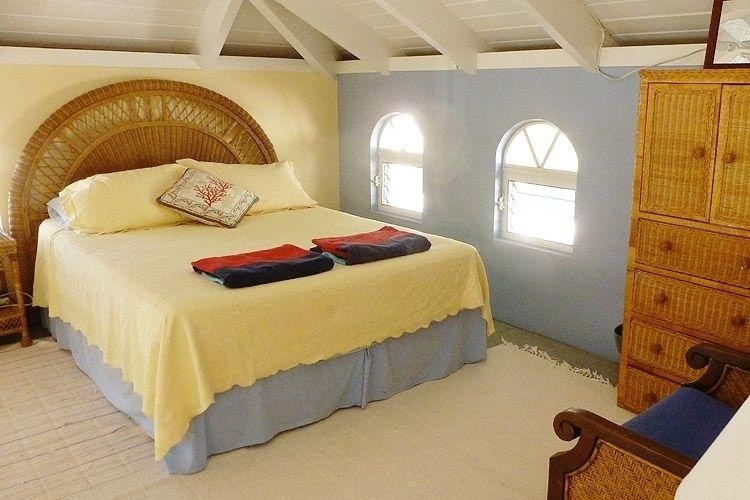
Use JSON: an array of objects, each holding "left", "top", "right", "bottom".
[{"left": 9, "top": 76, "right": 494, "bottom": 480}]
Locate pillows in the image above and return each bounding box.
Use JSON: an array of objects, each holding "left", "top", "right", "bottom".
[
  {"left": 175, "top": 156, "right": 317, "bottom": 217},
  {"left": 44, "top": 196, "right": 69, "bottom": 227},
  {"left": 58, "top": 162, "right": 197, "bottom": 236},
  {"left": 156, "top": 168, "right": 260, "bottom": 229}
]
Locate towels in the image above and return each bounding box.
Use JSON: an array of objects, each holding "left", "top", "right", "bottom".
[
  {"left": 191, "top": 241, "right": 335, "bottom": 289},
  {"left": 310, "top": 223, "right": 433, "bottom": 267}
]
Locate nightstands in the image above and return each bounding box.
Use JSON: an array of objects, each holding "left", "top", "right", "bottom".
[{"left": 0, "top": 229, "right": 33, "bottom": 348}]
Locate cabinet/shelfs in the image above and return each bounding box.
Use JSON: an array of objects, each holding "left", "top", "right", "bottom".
[{"left": 614, "top": 64, "right": 750, "bottom": 415}]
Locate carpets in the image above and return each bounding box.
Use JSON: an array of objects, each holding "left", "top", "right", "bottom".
[{"left": 0, "top": 325, "right": 639, "bottom": 500}]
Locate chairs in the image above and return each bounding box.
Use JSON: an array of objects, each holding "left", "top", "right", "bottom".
[{"left": 543, "top": 341, "right": 750, "bottom": 499}]
